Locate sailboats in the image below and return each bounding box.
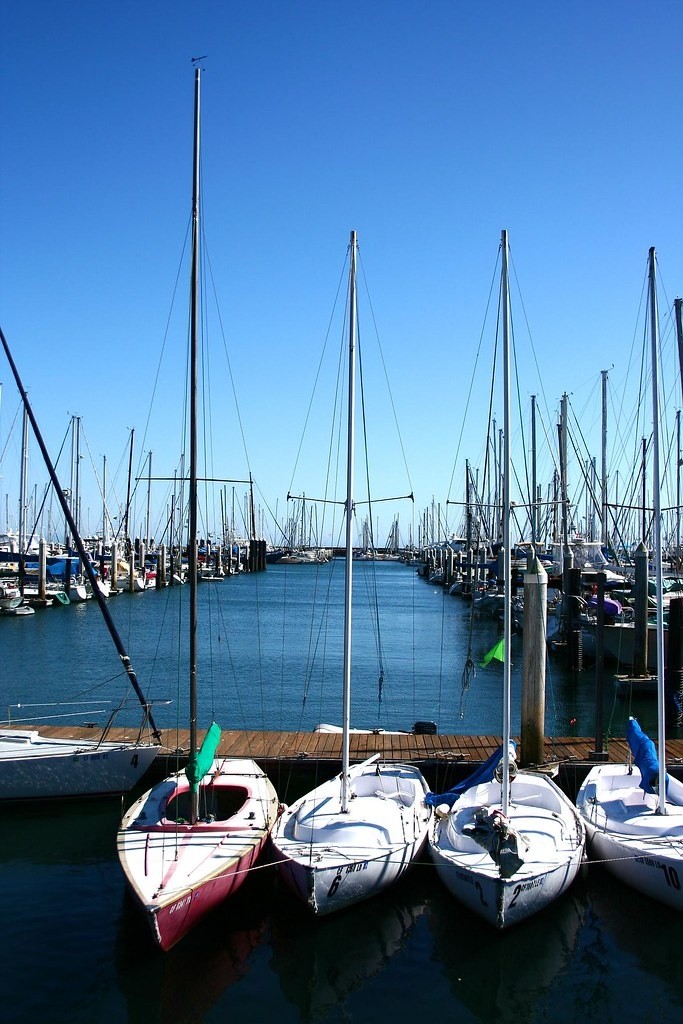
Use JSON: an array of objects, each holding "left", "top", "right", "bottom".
[
  {"left": 0, "top": 379, "right": 333, "bottom": 616},
  {"left": 359, "top": 296, "right": 683, "bottom": 701},
  {"left": 0, "top": 331, "right": 191, "bottom": 806},
  {"left": 569, "top": 245, "right": 683, "bottom": 915},
  {"left": 115, "top": 51, "right": 281, "bottom": 953},
  {"left": 429, "top": 228, "right": 586, "bottom": 933},
  {"left": 264, "top": 229, "right": 435, "bottom": 920}
]
